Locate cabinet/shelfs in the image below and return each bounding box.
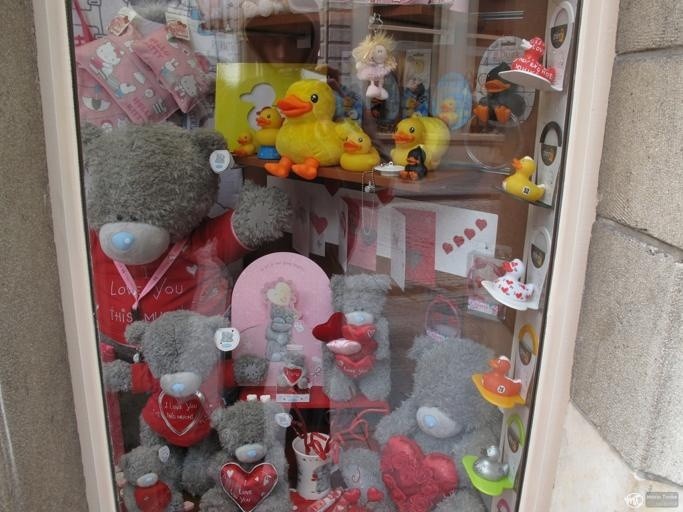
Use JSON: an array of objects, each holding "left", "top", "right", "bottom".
[{"left": 199, "top": 0, "right": 549, "bottom": 369}]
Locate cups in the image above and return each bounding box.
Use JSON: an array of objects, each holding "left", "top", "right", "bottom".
[{"left": 292, "top": 431, "right": 344, "bottom": 501}]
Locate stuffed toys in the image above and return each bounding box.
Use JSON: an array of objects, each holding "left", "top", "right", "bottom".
[
  {"left": 350, "top": 28, "right": 400, "bottom": 100},
  {"left": 278, "top": 353, "right": 310, "bottom": 392},
  {"left": 234, "top": 78, "right": 380, "bottom": 181},
  {"left": 397, "top": 145, "right": 428, "bottom": 181},
  {"left": 481, "top": 355, "right": 522, "bottom": 398},
  {"left": 435, "top": 97, "right": 458, "bottom": 123},
  {"left": 469, "top": 445, "right": 510, "bottom": 480},
  {"left": 503, "top": 156, "right": 548, "bottom": 203},
  {"left": 186, "top": 401, "right": 292, "bottom": 512},
  {"left": 339, "top": 329, "right": 512, "bottom": 512},
  {"left": 464, "top": 62, "right": 526, "bottom": 125},
  {"left": 494, "top": 258, "right": 537, "bottom": 302},
  {"left": 116, "top": 444, "right": 185, "bottom": 512},
  {"left": 510, "top": 36, "right": 558, "bottom": 84},
  {"left": 320, "top": 271, "right": 394, "bottom": 401},
  {"left": 391, "top": 114, "right": 451, "bottom": 172},
  {"left": 101, "top": 308, "right": 270, "bottom": 497},
  {"left": 81, "top": 120, "right": 298, "bottom": 460}
]
